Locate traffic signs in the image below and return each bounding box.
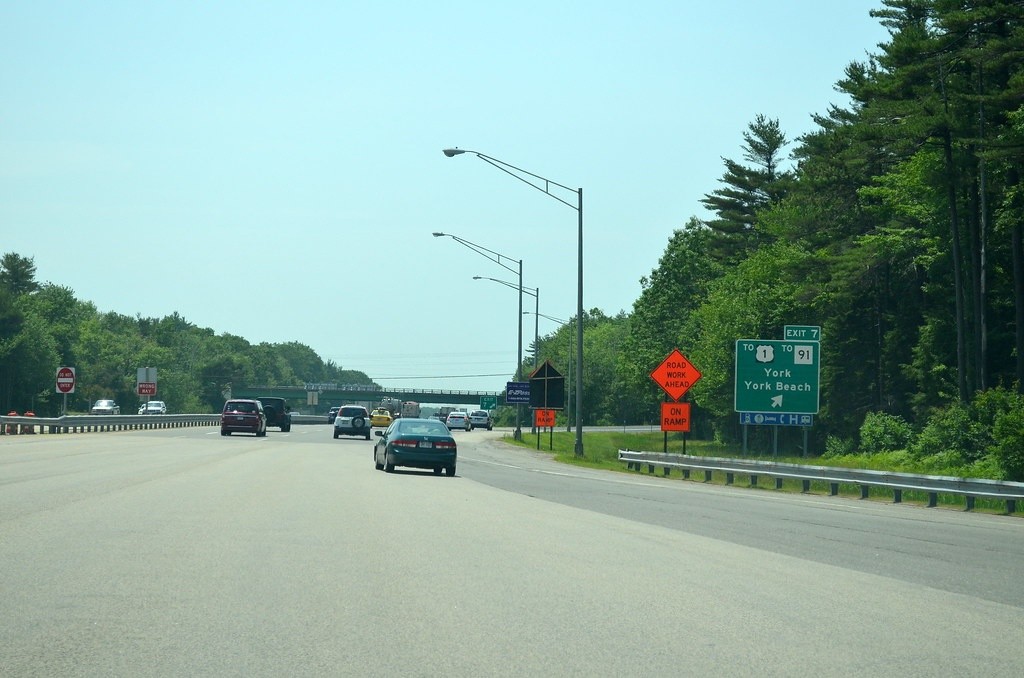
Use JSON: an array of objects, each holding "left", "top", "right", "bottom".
[
  {"left": 734, "top": 338, "right": 821, "bottom": 415},
  {"left": 480, "top": 396, "right": 497, "bottom": 410}
]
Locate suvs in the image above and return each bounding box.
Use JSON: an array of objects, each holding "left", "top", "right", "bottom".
[
  {"left": 257, "top": 398, "right": 291, "bottom": 432},
  {"left": 333, "top": 406, "right": 371, "bottom": 440}
]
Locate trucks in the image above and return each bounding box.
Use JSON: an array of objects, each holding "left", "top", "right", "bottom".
[
  {"left": 440, "top": 406, "right": 458, "bottom": 417},
  {"left": 401, "top": 402, "right": 421, "bottom": 418}
]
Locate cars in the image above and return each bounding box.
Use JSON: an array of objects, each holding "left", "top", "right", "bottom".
[
  {"left": 328, "top": 407, "right": 339, "bottom": 424},
  {"left": 429, "top": 412, "right": 471, "bottom": 432},
  {"left": 374, "top": 419, "right": 457, "bottom": 477},
  {"left": 138, "top": 401, "right": 166, "bottom": 415},
  {"left": 469, "top": 410, "right": 494, "bottom": 431},
  {"left": 221, "top": 400, "right": 267, "bottom": 436},
  {"left": 92, "top": 400, "right": 121, "bottom": 415},
  {"left": 371, "top": 410, "right": 392, "bottom": 428},
  {"left": 290, "top": 412, "right": 300, "bottom": 416}
]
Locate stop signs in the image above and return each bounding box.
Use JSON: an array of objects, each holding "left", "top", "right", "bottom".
[{"left": 57, "top": 368, "right": 74, "bottom": 392}]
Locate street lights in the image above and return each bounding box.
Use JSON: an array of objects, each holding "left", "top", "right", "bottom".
[
  {"left": 523, "top": 312, "right": 573, "bottom": 433},
  {"left": 433, "top": 233, "right": 522, "bottom": 442},
  {"left": 444, "top": 148, "right": 584, "bottom": 459},
  {"left": 472, "top": 276, "right": 539, "bottom": 434}
]
